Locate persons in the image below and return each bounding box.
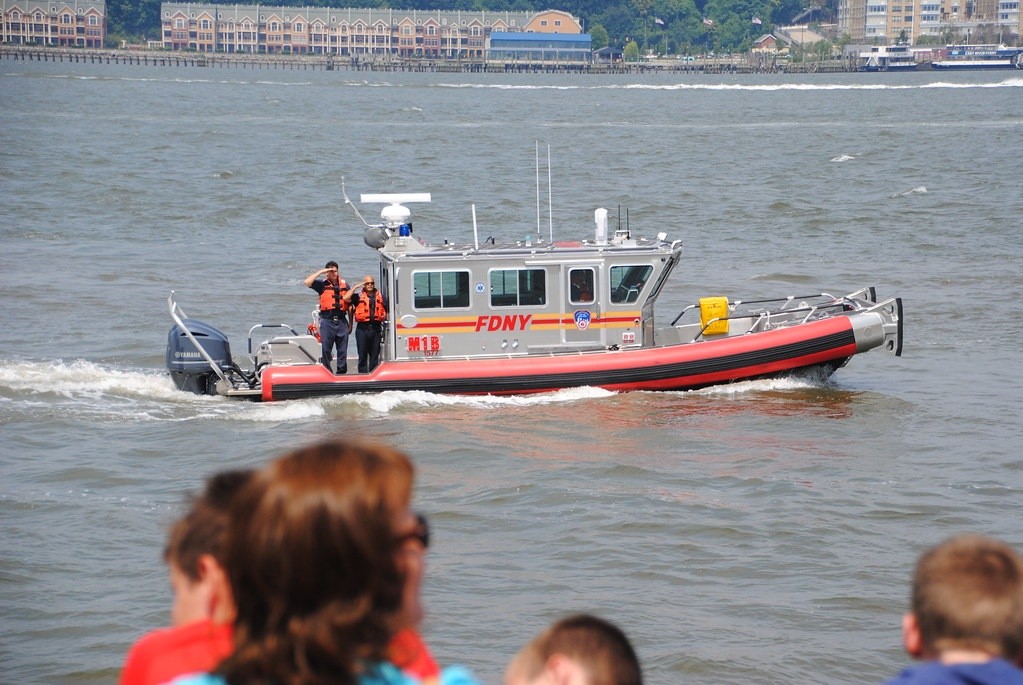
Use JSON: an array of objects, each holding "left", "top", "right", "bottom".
[
  {"left": 305, "top": 260, "right": 353, "bottom": 375},
  {"left": 167, "top": 442, "right": 481, "bottom": 685},
  {"left": 119, "top": 470, "right": 442, "bottom": 685},
  {"left": 343, "top": 276, "right": 386, "bottom": 373},
  {"left": 570, "top": 272, "right": 592, "bottom": 302},
  {"left": 891, "top": 536, "right": 1023, "bottom": 685},
  {"left": 506, "top": 614, "right": 644, "bottom": 685}
]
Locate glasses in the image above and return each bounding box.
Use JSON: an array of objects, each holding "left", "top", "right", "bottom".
[
  {"left": 365, "top": 282, "right": 374, "bottom": 285},
  {"left": 395, "top": 513, "right": 429, "bottom": 549}
]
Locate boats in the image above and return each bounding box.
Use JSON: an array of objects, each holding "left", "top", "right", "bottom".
[
  {"left": 167, "top": 139, "right": 903, "bottom": 402},
  {"left": 857, "top": 45, "right": 918, "bottom": 72},
  {"left": 931, "top": 42, "right": 1023, "bottom": 71}
]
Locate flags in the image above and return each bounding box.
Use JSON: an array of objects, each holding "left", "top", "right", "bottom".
[
  {"left": 752, "top": 16, "right": 761, "bottom": 24},
  {"left": 656, "top": 17, "right": 664, "bottom": 25},
  {"left": 704, "top": 17, "right": 712, "bottom": 25}
]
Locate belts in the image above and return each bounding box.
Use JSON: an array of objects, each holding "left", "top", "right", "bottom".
[{"left": 321, "top": 315, "right": 345, "bottom": 321}]
